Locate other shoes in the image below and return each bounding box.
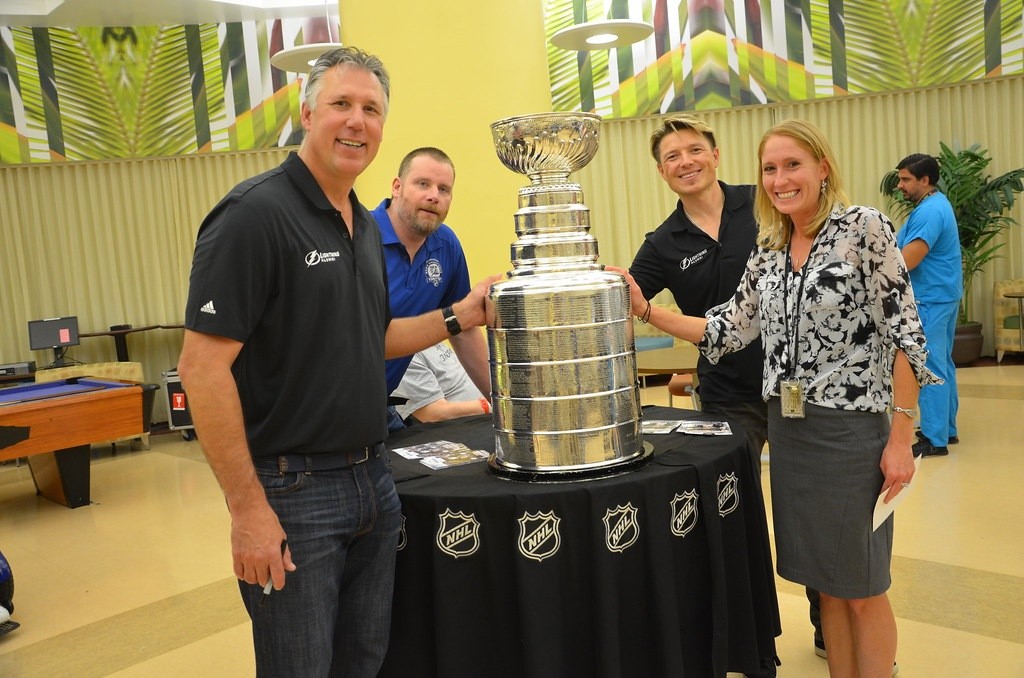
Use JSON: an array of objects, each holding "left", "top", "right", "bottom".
[
  {"left": 912, "top": 439, "right": 948, "bottom": 457},
  {"left": 915, "top": 431, "right": 959, "bottom": 444},
  {"left": 815, "top": 637, "right": 898, "bottom": 675}
]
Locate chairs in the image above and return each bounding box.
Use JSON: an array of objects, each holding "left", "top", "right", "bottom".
[
  {"left": 631, "top": 303, "right": 693, "bottom": 388},
  {"left": 993, "top": 279, "right": 1024, "bottom": 365}
]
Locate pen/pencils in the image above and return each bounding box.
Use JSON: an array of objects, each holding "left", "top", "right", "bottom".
[{"left": 259, "top": 539, "right": 287, "bottom": 608}]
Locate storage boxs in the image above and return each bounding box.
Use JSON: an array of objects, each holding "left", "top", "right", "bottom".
[{"left": 161, "top": 368, "right": 196, "bottom": 441}]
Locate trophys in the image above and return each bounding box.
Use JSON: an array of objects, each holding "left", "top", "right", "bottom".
[{"left": 484, "top": 112, "right": 655, "bottom": 482}]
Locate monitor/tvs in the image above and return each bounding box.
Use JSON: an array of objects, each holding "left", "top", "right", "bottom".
[{"left": 28, "top": 315, "right": 81, "bottom": 370}]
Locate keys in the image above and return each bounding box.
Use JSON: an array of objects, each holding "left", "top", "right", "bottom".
[{"left": 789, "top": 385, "right": 798, "bottom": 409}]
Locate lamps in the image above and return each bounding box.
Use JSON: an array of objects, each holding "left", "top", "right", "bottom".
[
  {"left": 548, "top": 0, "right": 654, "bottom": 52},
  {"left": 269, "top": 0, "right": 342, "bottom": 74}
]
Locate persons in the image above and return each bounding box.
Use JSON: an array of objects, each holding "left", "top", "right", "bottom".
[
  {"left": 604, "top": 119, "right": 945, "bottom": 678},
  {"left": 367, "top": 148, "right": 496, "bottom": 395},
  {"left": 176, "top": 46, "right": 504, "bottom": 678},
  {"left": 628, "top": 112, "right": 899, "bottom": 676},
  {"left": 895, "top": 153, "right": 963, "bottom": 458},
  {"left": 390, "top": 341, "right": 493, "bottom": 424}
]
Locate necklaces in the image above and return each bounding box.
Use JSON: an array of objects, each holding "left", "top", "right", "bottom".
[{"left": 792, "top": 251, "right": 808, "bottom": 267}]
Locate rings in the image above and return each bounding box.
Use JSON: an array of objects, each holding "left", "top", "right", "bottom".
[{"left": 902, "top": 483, "right": 909, "bottom": 488}]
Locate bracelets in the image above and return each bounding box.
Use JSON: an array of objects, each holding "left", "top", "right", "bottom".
[
  {"left": 638, "top": 301, "right": 651, "bottom": 324},
  {"left": 892, "top": 406, "right": 918, "bottom": 418},
  {"left": 442, "top": 305, "right": 462, "bottom": 336},
  {"left": 480, "top": 398, "right": 489, "bottom": 414}
]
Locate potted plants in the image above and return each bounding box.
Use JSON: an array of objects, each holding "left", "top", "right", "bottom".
[{"left": 878, "top": 139, "right": 1024, "bottom": 370}]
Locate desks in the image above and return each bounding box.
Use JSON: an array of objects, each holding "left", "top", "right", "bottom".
[
  {"left": 384, "top": 405, "right": 782, "bottom": 678},
  {"left": 161, "top": 323, "right": 184, "bottom": 330},
  {"left": 78, "top": 324, "right": 161, "bottom": 362},
  {"left": 636, "top": 346, "right": 700, "bottom": 411},
  {"left": 0, "top": 376, "right": 144, "bottom": 508},
  {"left": 1003, "top": 292, "right": 1024, "bottom": 350}
]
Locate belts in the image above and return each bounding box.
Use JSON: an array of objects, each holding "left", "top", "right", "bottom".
[{"left": 279, "top": 443, "right": 380, "bottom": 472}]
